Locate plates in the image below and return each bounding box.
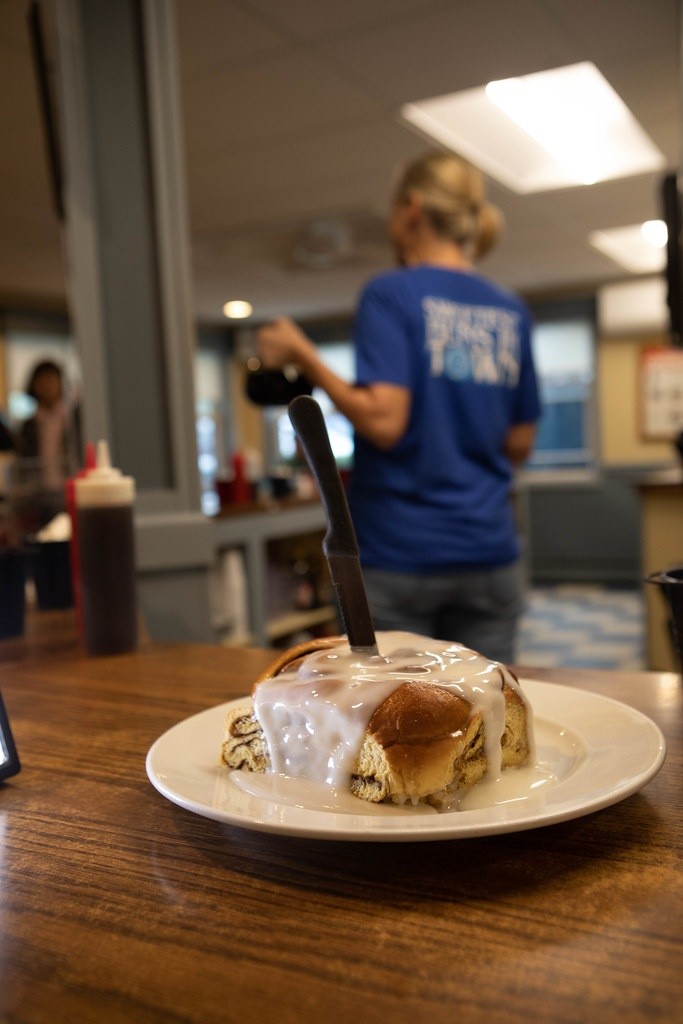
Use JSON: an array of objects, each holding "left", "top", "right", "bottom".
[{"left": 145, "top": 678, "right": 668, "bottom": 842}]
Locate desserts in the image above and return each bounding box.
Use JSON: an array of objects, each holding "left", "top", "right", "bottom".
[{"left": 226, "top": 630, "right": 535, "bottom": 803}]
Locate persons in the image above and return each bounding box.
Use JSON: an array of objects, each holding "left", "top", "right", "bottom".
[
  {"left": 257, "top": 153, "right": 542, "bottom": 669},
  {"left": 15, "top": 360, "right": 82, "bottom": 488}
]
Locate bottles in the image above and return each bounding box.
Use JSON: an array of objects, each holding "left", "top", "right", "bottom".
[
  {"left": 75, "top": 440, "right": 138, "bottom": 654},
  {"left": 63, "top": 444, "right": 95, "bottom": 639}
]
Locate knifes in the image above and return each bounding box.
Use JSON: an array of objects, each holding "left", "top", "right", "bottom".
[{"left": 288, "top": 397, "right": 379, "bottom": 655}]
